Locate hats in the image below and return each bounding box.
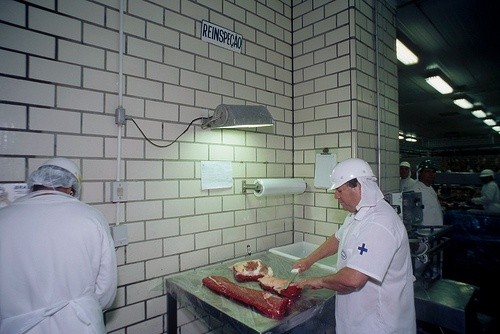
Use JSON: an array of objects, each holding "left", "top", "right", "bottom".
[
  {"left": 39, "top": 157, "right": 81, "bottom": 184},
  {"left": 400, "top": 162, "right": 410, "bottom": 168},
  {"left": 480, "top": 169, "right": 494, "bottom": 179},
  {"left": 418, "top": 160, "right": 435, "bottom": 169},
  {"left": 330, "top": 158, "right": 376, "bottom": 190}
]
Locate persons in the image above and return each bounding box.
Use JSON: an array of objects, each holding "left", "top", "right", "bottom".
[
  {"left": 0, "top": 157, "right": 118, "bottom": 334},
  {"left": 470, "top": 168, "right": 500, "bottom": 213},
  {"left": 289, "top": 159, "right": 417, "bottom": 334},
  {"left": 400, "top": 161, "right": 444, "bottom": 283}
]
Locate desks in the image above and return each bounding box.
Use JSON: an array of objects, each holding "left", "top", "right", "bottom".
[{"left": 166, "top": 250, "right": 336, "bottom": 334}]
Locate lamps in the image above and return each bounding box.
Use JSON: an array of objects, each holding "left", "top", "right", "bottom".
[
  {"left": 398, "top": 128, "right": 418, "bottom": 143},
  {"left": 200, "top": 104, "right": 274, "bottom": 130},
  {"left": 396, "top": 35, "right": 500, "bottom": 137}
]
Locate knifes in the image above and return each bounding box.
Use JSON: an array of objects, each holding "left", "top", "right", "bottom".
[{"left": 284, "top": 267, "right": 301, "bottom": 289}]
